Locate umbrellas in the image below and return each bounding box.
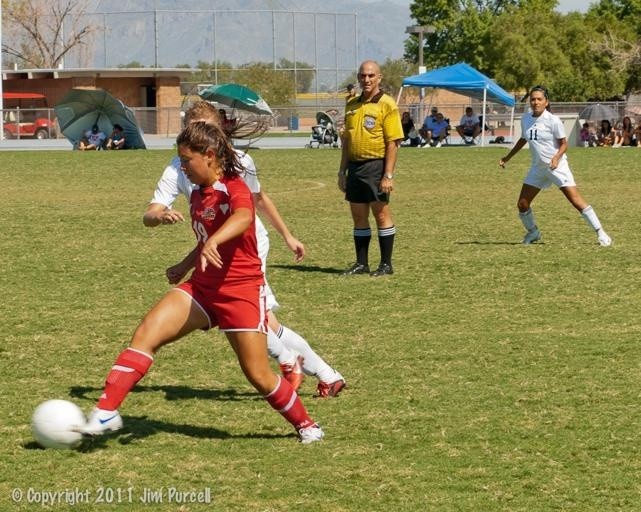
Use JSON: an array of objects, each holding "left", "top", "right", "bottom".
[
  {"left": 200, "top": 84, "right": 274, "bottom": 121},
  {"left": 57, "top": 85, "right": 146, "bottom": 149},
  {"left": 578, "top": 101, "right": 620, "bottom": 121}
]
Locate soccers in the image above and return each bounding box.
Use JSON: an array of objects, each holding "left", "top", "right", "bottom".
[{"left": 31, "top": 401, "right": 83, "bottom": 450}]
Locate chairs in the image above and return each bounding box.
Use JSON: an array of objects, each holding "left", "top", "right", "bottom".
[
  {"left": 454, "top": 115, "right": 482, "bottom": 145},
  {"left": 418, "top": 118, "right": 450, "bottom": 147}
]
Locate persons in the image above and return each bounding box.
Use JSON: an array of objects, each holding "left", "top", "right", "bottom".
[
  {"left": 218, "top": 109, "right": 228, "bottom": 120},
  {"left": 87, "top": 122, "right": 319, "bottom": 449},
  {"left": 78, "top": 125, "right": 104, "bottom": 151},
  {"left": 498, "top": 80, "right": 616, "bottom": 249},
  {"left": 580, "top": 117, "right": 641, "bottom": 148},
  {"left": 139, "top": 102, "right": 348, "bottom": 404},
  {"left": 103, "top": 124, "right": 126, "bottom": 150},
  {"left": 399, "top": 106, "right": 483, "bottom": 149},
  {"left": 337, "top": 62, "right": 400, "bottom": 277}
]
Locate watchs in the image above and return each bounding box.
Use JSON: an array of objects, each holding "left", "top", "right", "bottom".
[{"left": 382, "top": 171, "right": 394, "bottom": 180}]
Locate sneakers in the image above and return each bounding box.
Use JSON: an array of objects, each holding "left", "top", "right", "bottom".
[
  {"left": 370, "top": 262, "right": 392, "bottom": 276},
  {"left": 277, "top": 354, "right": 347, "bottom": 444},
  {"left": 436, "top": 142, "right": 441, "bottom": 148},
  {"left": 82, "top": 406, "right": 124, "bottom": 435},
  {"left": 339, "top": 263, "right": 369, "bottom": 276},
  {"left": 597, "top": 233, "right": 611, "bottom": 246},
  {"left": 422, "top": 143, "right": 431, "bottom": 148},
  {"left": 522, "top": 229, "right": 541, "bottom": 244}
]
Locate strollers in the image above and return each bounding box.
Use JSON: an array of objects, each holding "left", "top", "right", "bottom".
[{"left": 306, "top": 108, "right": 338, "bottom": 149}]
